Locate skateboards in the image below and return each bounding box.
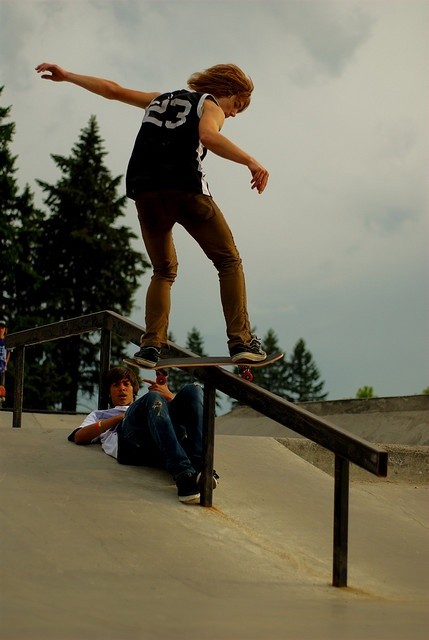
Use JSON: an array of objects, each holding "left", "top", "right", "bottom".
[{"left": 123, "top": 352, "right": 284, "bottom": 385}]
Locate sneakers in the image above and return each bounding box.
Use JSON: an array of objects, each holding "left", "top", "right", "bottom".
[
  {"left": 227, "top": 335, "right": 267, "bottom": 363},
  {"left": 133, "top": 347, "right": 160, "bottom": 368},
  {"left": 176, "top": 470, "right": 217, "bottom": 503}
]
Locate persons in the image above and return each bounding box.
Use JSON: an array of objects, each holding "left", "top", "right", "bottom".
[
  {"left": 0, "top": 320, "right": 14, "bottom": 408},
  {"left": 35, "top": 62, "right": 270, "bottom": 367},
  {"left": 68, "top": 366, "right": 219, "bottom": 502}
]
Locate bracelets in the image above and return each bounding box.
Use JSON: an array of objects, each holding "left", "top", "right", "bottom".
[
  {"left": 99, "top": 421, "right": 104, "bottom": 433},
  {"left": 96, "top": 422, "right": 101, "bottom": 433}
]
